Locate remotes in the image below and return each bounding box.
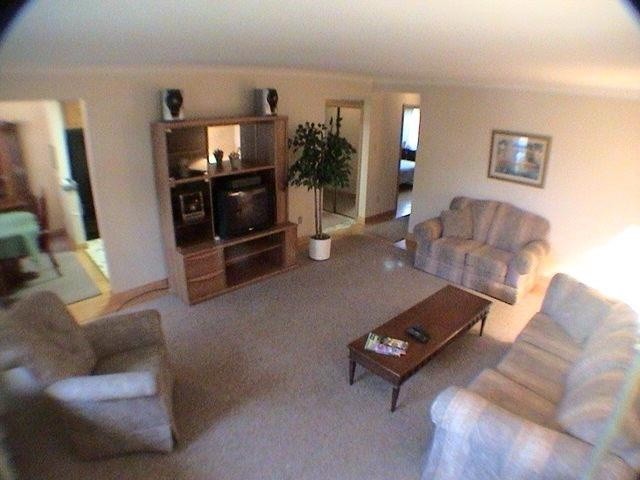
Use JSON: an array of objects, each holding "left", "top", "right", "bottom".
[
  {"left": 407, "top": 328, "right": 428, "bottom": 342},
  {"left": 415, "top": 325, "right": 428, "bottom": 336}
]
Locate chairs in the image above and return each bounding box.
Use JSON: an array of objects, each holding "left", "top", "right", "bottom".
[
  {"left": 0, "top": 290, "right": 180, "bottom": 458},
  {"left": 22, "top": 197, "right": 66, "bottom": 276}
]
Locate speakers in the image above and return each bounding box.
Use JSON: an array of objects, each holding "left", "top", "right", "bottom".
[
  {"left": 160, "top": 87, "right": 184, "bottom": 121},
  {"left": 255, "top": 88, "right": 279, "bottom": 115}
]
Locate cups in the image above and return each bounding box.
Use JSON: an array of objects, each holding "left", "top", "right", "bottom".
[{"left": 231, "top": 158, "right": 241, "bottom": 168}]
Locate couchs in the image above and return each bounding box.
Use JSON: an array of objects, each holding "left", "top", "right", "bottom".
[
  {"left": 420, "top": 273, "right": 640, "bottom": 480},
  {"left": 414, "top": 196, "right": 550, "bottom": 306}
]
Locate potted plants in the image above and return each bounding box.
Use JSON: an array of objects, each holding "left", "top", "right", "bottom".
[{"left": 286, "top": 118, "right": 354, "bottom": 262}]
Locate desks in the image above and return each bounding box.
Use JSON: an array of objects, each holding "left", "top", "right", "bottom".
[{"left": 0, "top": 211, "right": 43, "bottom": 283}]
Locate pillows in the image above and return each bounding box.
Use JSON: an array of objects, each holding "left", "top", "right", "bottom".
[{"left": 440, "top": 207, "right": 473, "bottom": 239}]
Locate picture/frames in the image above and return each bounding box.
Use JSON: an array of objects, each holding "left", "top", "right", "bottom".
[{"left": 487, "top": 129, "right": 551, "bottom": 188}]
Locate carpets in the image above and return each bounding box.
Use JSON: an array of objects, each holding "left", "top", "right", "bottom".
[{"left": 1, "top": 251, "right": 101, "bottom": 305}]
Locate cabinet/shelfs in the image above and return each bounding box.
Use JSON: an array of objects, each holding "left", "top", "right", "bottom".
[{"left": 150, "top": 114, "right": 295, "bottom": 307}]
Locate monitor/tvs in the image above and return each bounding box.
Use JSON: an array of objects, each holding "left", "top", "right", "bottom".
[{"left": 214, "top": 184, "right": 274, "bottom": 239}]
